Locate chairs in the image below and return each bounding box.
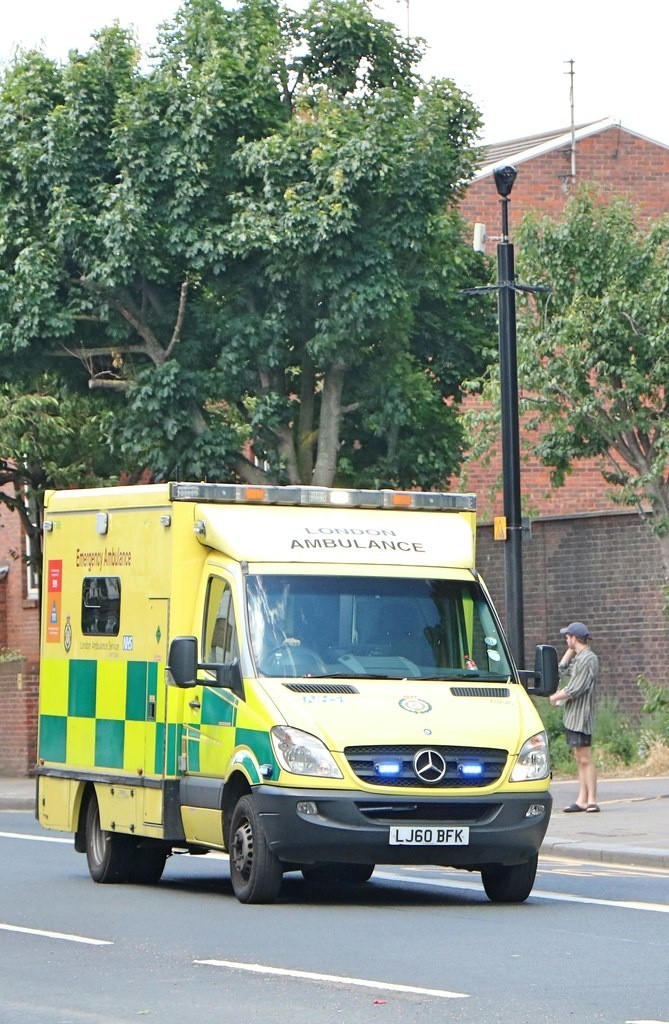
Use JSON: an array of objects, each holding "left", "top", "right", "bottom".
[{"left": 366, "top": 604, "right": 434, "bottom": 667}]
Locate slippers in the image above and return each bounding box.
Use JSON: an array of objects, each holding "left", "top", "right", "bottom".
[
  {"left": 563, "top": 803, "right": 586, "bottom": 811},
  {"left": 587, "top": 804, "right": 600, "bottom": 812}
]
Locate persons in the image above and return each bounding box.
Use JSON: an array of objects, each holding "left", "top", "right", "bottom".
[
  {"left": 550, "top": 622, "right": 600, "bottom": 812},
  {"left": 252, "top": 599, "right": 306, "bottom": 676}
]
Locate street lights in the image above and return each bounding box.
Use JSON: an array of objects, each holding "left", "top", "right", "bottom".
[{"left": 456, "top": 164, "right": 552, "bottom": 693}]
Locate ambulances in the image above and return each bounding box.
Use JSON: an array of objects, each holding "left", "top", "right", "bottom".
[{"left": 34, "top": 481, "right": 553, "bottom": 903}]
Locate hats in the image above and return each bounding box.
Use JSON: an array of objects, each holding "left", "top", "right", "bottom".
[{"left": 559, "top": 621, "right": 591, "bottom": 640}]
